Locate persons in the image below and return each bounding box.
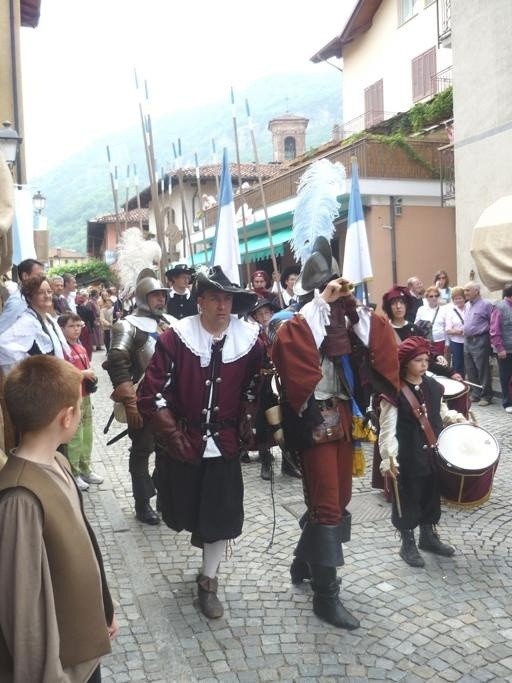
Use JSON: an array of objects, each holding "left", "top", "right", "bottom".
[
  {"left": 1, "top": 354, "right": 118, "bottom": 682},
  {"left": 2, "top": 256, "right": 512, "bottom": 522},
  {"left": 136, "top": 266, "right": 267, "bottom": 618},
  {"left": 378, "top": 336, "right": 456, "bottom": 567},
  {"left": 271, "top": 238, "right": 402, "bottom": 627}
]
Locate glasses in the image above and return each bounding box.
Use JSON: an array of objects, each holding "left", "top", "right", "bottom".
[
  {"left": 430, "top": 295, "right": 441, "bottom": 298},
  {"left": 437, "top": 277, "right": 446, "bottom": 281},
  {"left": 35, "top": 288, "right": 55, "bottom": 296}
]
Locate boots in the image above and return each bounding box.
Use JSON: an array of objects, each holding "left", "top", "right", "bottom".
[
  {"left": 289, "top": 507, "right": 352, "bottom": 585},
  {"left": 281, "top": 447, "right": 303, "bottom": 477},
  {"left": 257, "top": 440, "right": 275, "bottom": 480},
  {"left": 292, "top": 519, "right": 362, "bottom": 630},
  {"left": 418, "top": 522, "right": 455, "bottom": 556},
  {"left": 398, "top": 527, "right": 427, "bottom": 567}
]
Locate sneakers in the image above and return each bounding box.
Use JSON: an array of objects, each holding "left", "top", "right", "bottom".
[
  {"left": 72, "top": 475, "right": 91, "bottom": 491},
  {"left": 469, "top": 396, "right": 492, "bottom": 406},
  {"left": 80, "top": 472, "right": 104, "bottom": 484},
  {"left": 506, "top": 407, "right": 512, "bottom": 413}
]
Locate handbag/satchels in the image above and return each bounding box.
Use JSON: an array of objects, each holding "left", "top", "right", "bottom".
[
  {"left": 308, "top": 396, "right": 346, "bottom": 447},
  {"left": 113, "top": 383, "right": 138, "bottom": 424}
]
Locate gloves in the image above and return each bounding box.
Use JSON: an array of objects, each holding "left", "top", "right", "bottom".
[
  {"left": 110, "top": 380, "right": 144, "bottom": 430},
  {"left": 322, "top": 297, "right": 353, "bottom": 358},
  {"left": 238, "top": 398, "right": 259, "bottom": 454},
  {"left": 146, "top": 407, "right": 193, "bottom": 463}
]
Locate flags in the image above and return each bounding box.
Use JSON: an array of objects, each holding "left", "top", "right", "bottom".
[
  {"left": 211, "top": 147, "right": 243, "bottom": 287},
  {"left": 340, "top": 160, "right": 375, "bottom": 305}
]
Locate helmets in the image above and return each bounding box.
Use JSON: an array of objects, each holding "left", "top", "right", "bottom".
[
  {"left": 134, "top": 268, "right": 172, "bottom": 317},
  {"left": 291, "top": 235, "right": 341, "bottom": 295}
]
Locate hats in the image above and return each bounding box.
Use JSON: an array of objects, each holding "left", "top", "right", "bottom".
[
  {"left": 166, "top": 264, "right": 196, "bottom": 284},
  {"left": 250, "top": 270, "right": 269, "bottom": 282},
  {"left": 189, "top": 265, "right": 257, "bottom": 316},
  {"left": 397, "top": 335, "right": 435, "bottom": 369},
  {"left": 381, "top": 285, "right": 419, "bottom": 315},
  {"left": 280, "top": 265, "right": 301, "bottom": 290},
  {"left": 248, "top": 291, "right": 273, "bottom": 314}
]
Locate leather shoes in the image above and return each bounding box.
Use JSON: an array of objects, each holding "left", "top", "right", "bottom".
[
  {"left": 196, "top": 573, "right": 224, "bottom": 619},
  {"left": 241, "top": 452, "right": 250, "bottom": 463},
  {"left": 134, "top": 503, "right": 161, "bottom": 525},
  {"left": 259, "top": 451, "right": 275, "bottom": 461}
]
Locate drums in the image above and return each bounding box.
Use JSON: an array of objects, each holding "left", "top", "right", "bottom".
[
  {"left": 431, "top": 374, "right": 474, "bottom": 421},
  {"left": 433, "top": 421, "right": 500, "bottom": 509}
]
